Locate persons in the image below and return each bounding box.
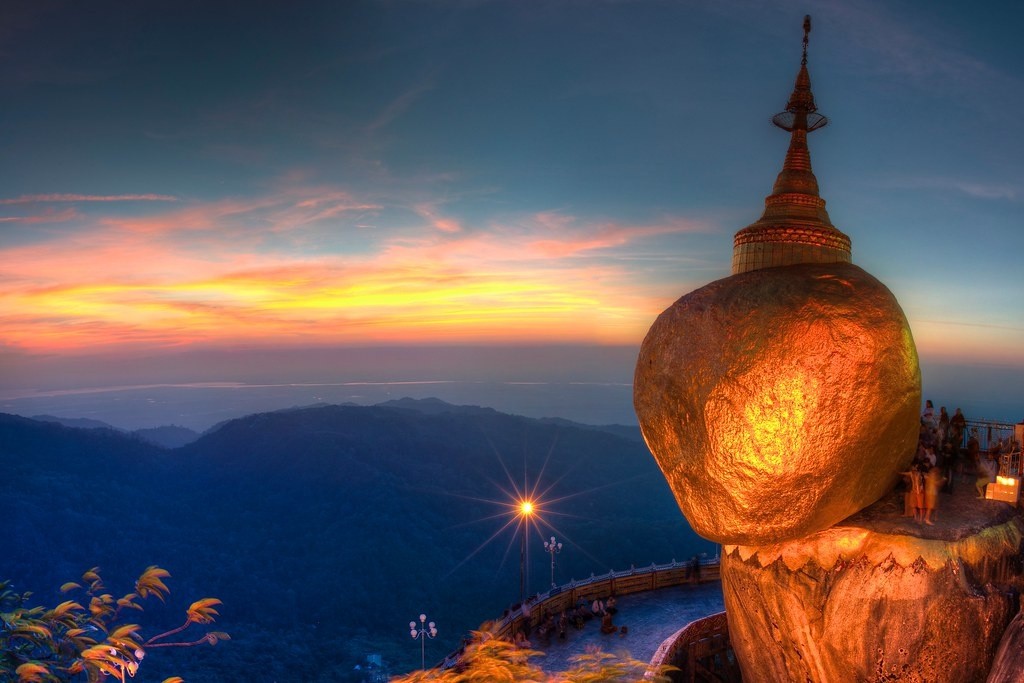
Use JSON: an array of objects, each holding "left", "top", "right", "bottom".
[
  {"left": 689, "top": 553, "right": 701, "bottom": 585},
  {"left": 515, "top": 592, "right": 619, "bottom": 650},
  {"left": 897, "top": 399, "right": 1022, "bottom": 527}
]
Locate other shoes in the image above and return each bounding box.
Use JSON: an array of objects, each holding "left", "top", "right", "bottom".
[
  {"left": 976, "top": 496, "right": 985, "bottom": 499},
  {"left": 912, "top": 518, "right": 941, "bottom": 526}
]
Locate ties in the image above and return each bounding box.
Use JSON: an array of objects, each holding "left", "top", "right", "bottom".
[{"left": 598, "top": 601, "right": 600, "bottom": 610}]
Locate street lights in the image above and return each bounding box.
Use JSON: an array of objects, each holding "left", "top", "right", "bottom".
[
  {"left": 410, "top": 615, "right": 438, "bottom": 670},
  {"left": 543, "top": 535, "right": 562, "bottom": 588},
  {"left": 521, "top": 501, "right": 533, "bottom": 605}
]
